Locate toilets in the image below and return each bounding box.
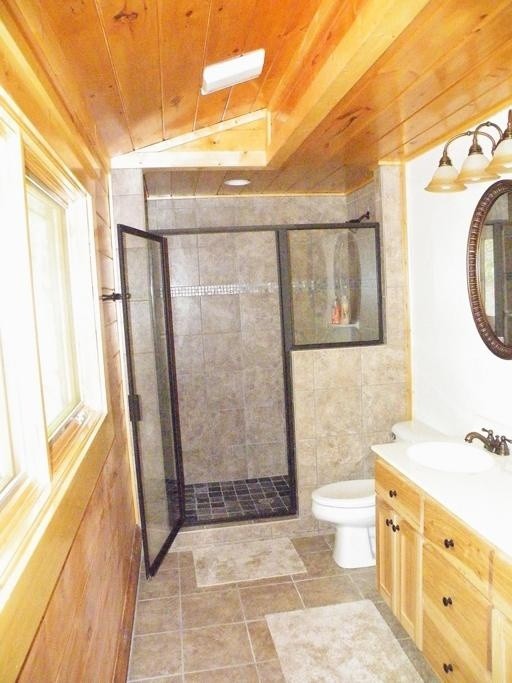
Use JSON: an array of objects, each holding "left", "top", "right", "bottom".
[{"left": 309, "top": 420, "right": 454, "bottom": 569}]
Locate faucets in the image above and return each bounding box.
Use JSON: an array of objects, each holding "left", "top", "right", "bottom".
[{"left": 465, "top": 427, "right": 512, "bottom": 455}]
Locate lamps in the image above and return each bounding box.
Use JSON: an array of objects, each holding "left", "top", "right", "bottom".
[
  {"left": 422, "top": 108, "right": 512, "bottom": 195},
  {"left": 200, "top": 47, "right": 267, "bottom": 98}
]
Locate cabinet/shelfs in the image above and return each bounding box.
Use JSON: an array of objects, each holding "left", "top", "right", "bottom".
[{"left": 372, "top": 458, "right": 511, "bottom": 683}]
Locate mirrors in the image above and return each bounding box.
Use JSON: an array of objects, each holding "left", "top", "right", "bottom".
[{"left": 463, "top": 178, "right": 512, "bottom": 362}]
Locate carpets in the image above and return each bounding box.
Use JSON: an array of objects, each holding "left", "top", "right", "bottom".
[{"left": 192, "top": 537, "right": 424, "bottom": 682}]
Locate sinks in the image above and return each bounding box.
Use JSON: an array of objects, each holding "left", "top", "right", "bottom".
[{"left": 404, "top": 440, "right": 493, "bottom": 476}]
